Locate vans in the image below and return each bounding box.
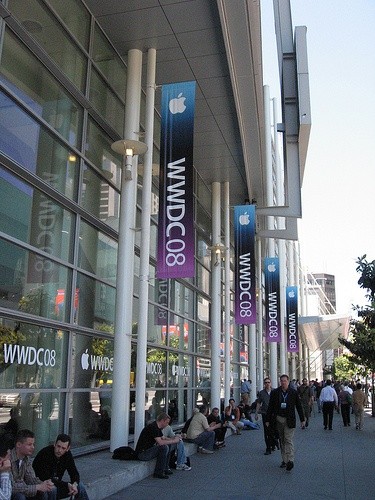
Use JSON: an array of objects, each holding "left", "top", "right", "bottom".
[{"left": 96, "top": 371, "right": 134, "bottom": 390}]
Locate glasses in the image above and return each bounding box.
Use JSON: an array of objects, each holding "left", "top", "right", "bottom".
[{"left": 265, "top": 382, "right": 270, "bottom": 383}]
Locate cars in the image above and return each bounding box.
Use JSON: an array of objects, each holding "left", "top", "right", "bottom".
[{"left": 0, "top": 381, "right": 43, "bottom": 409}]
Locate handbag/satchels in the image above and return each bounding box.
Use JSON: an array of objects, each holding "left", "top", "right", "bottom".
[{"left": 345, "top": 391, "right": 352, "bottom": 403}]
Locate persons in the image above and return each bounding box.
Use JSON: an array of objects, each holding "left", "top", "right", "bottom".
[
  {"left": 320, "top": 380, "right": 338, "bottom": 431},
  {"left": 352, "top": 383, "right": 368, "bottom": 430},
  {"left": 0, "top": 429, "right": 90, "bottom": 500},
  {"left": 338, "top": 384, "right": 352, "bottom": 427},
  {"left": 250, "top": 400, "right": 261, "bottom": 414},
  {"left": 297, "top": 378, "right": 313, "bottom": 429},
  {"left": 237, "top": 393, "right": 261, "bottom": 430},
  {"left": 180, "top": 405, "right": 227, "bottom": 454},
  {"left": 308, "top": 378, "right": 327, "bottom": 413},
  {"left": 330, "top": 378, "right": 361, "bottom": 414},
  {"left": 225, "top": 399, "right": 244, "bottom": 434},
  {"left": 6, "top": 375, "right": 211, "bottom": 447},
  {"left": 289, "top": 378, "right": 302, "bottom": 391},
  {"left": 265, "top": 374, "right": 306, "bottom": 472},
  {"left": 255, "top": 378, "right": 276, "bottom": 456},
  {"left": 135, "top": 412, "right": 192, "bottom": 477},
  {"left": 241, "top": 379, "right": 252, "bottom": 405}
]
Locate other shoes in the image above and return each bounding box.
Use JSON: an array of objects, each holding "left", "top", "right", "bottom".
[
  {"left": 329, "top": 426, "right": 332, "bottom": 430},
  {"left": 280, "top": 461, "right": 286, "bottom": 468},
  {"left": 264, "top": 449, "right": 271, "bottom": 455},
  {"left": 198, "top": 446, "right": 203, "bottom": 452},
  {"left": 202, "top": 448, "right": 214, "bottom": 454},
  {"left": 358, "top": 425, "right": 362, "bottom": 429},
  {"left": 153, "top": 473, "right": 169, "bottom": 479},
  {"left": 324, "top": 426, "right": 327, "bottom": 429},
  {"left": 286, "top": 461, "right": 294, "bottom": 472},
  {"left": 305, "top": 420, "right": 309, "bottom": 427},
  {"left": 215, "top": 441, "right": 225, "bottom": 446},
  {"left": 302, "top": 426, "right": 305, "bottom": 428},
  {"left": 165, "top": 470, "right": 173, "bottom": 474},
  {"left": 348, "top": 422, "right": 351, "bottom": 426},
  {"left": 175, "top": 464, "right": 191, "bottom": 470},
  {"left": 344, "top": 423, "right": 347, "bottom": 426},
  {"left": 355, "top": 423, "right": 359, "bottom": 429}
]
